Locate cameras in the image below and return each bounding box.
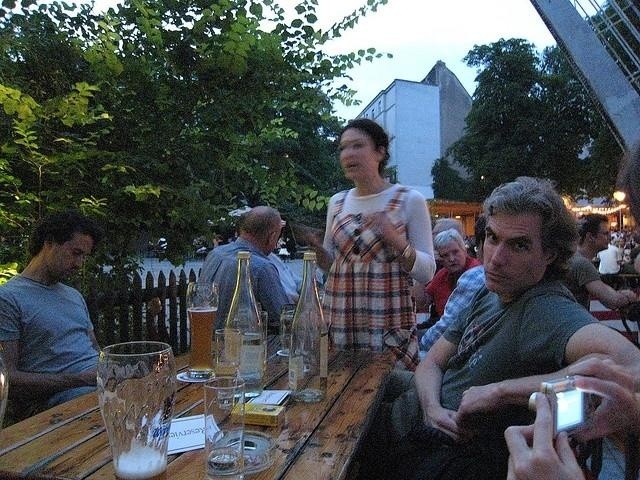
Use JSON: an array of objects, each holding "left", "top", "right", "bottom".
[{"left": 528, "top": 376, "right": 593, "bottom": 440}]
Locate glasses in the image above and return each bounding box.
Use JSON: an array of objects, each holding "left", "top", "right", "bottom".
[{"left": 585, "top": 230, "right": 610, "bottom": 236}]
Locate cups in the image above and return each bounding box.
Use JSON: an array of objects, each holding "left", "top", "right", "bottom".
[
  {"left": 277, "top": 311, "right": 293, "bottom": 359},
  {"left": 187, "top": 281, "right": 218, "bottom": 373},
  {"left": 96, "top": 340, "right": 178, "bottom": 477},
  {"left": 202, "top": 376, "right": 246, "bottom": 477},
  {"left": 214, "top": 329, "right": 242, "bottom": 377}
]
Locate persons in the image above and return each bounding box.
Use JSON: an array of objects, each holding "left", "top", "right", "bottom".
[
  {"left": 413, "top": 219, "right": 486, "bottom": 352},
  {"left": 563, "top": 213, "right": 640, "bottom": 312},
  {"left": 356, "top": 175, "right": 640, "bottom": 480},
  {"left": 194, "top": 203, "right": 292, "bottom": 343},
  {"left": 304, "top": 117, "right": 438, "bottom": 371},
  {"left": 501, "top": 136, "right": 640, "bottom": 480},
  {"left": 0, "top": 209, "right": 102, "bottom": 411},
  {"left": 271, "top": 253, "right": 301, "bottom": 304}
]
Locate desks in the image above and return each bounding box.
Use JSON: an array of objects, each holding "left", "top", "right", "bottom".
[{"left": 1, "top": 341, "right": 391, "bottom": 480}]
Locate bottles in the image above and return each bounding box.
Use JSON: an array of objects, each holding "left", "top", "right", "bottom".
[
  {"left": 224, "top": 251, "right": 265, "bottom": 398},
  {"left": 288, "top": 252, "right": 328, "bottom": 403}
]
